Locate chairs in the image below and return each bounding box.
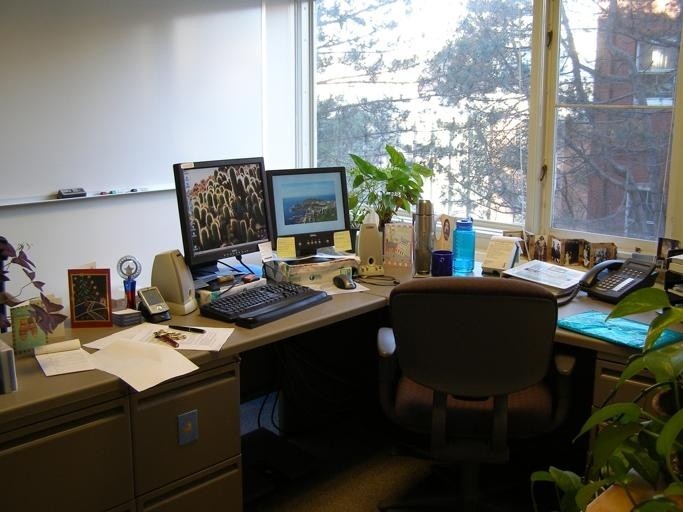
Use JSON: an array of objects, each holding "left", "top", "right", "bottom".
[{"left": 385, "top": 275, "right": 561, "bottom": 510}]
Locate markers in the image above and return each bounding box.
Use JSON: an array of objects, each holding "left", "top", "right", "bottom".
[
  {"left": 131, "top": 189, "right": 149, "bottom": 192},
  {"left": 100, "top": 190, "right": 117, "bottom": 195}
]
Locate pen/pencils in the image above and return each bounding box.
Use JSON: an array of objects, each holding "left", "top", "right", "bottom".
[{"left": 169, "top": 325, "right": 205, "bottom": 333}]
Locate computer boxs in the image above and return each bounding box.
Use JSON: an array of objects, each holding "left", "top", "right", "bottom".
[{"left": 264, "top": 252, "right": 356, "bottom": 285}]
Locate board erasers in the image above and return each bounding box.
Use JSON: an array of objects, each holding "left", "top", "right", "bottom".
[{"left": 57, "top": 188, "right": 87, "bottom": 199}]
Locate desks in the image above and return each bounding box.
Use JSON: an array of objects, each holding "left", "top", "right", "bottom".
[
  {"left": 0, "top": 243, "right": 679, "bottom": 511},
  {"left": 584, "top": 455, "right": 682, "bottom": 512}
]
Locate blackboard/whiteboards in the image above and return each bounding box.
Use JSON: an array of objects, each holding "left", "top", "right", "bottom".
[{"left": 0, "top": 0, "right": 268, "bottom": 208}]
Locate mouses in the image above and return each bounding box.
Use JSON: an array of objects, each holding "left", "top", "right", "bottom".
[{"left": 333, "top": 275, "right": 356, "bottom": 289}]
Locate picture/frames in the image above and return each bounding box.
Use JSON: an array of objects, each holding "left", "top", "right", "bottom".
[
  {"left": 68, "top": 267, "right": 113, "bottom": 330},
  {"left": 502, "top": 230, "right": 617, "bottom": 270}
]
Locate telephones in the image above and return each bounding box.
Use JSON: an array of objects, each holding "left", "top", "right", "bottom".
[{"left": 576, "top": 257, "right": 659, "bottom": 305}]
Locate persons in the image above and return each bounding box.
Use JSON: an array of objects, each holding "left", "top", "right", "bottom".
[
  {"left": 504, "top": 231, "right": 613, "bottom": 269},
  {"left": 441, "top": 219, "right": 451, "bottom": 251}
]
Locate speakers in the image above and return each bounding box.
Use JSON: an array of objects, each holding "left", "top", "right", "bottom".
[
  {"left": 355, "top": 223, "right": 385, "bottom": 278},
  {"left": 151, "top": 249, "right": 199, "bottom": 316}
]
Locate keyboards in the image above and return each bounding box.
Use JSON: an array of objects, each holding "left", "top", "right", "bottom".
[{"left": 199, "top": 281, "right": 333, "bottom": 329}]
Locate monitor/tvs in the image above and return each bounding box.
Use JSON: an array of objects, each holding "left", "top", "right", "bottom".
[
  {"left": 173, "top": 157, "right": 271, "bottom": 291},
  {"left": 265, "top": 166, "right": 351, "bottom": 263}
]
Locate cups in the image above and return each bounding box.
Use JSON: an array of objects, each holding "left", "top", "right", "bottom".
[{"left": 431, "top": 250, "right": 452, "bottom": 277}]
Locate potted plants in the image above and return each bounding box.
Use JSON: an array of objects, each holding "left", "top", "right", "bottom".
[
  {"left": 340, "top": 144, "right": 433, "bottom": 254},
  {"left": 527, "top": 283, "right": 683, "bottom": 510}
]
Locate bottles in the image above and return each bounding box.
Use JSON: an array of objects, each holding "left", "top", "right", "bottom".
[
  {"left": 413, "top": 199, "right": 434, "bottom": 275},
  {"left": 452, "top": 216, "right": 476, "bottom": 274}
]
